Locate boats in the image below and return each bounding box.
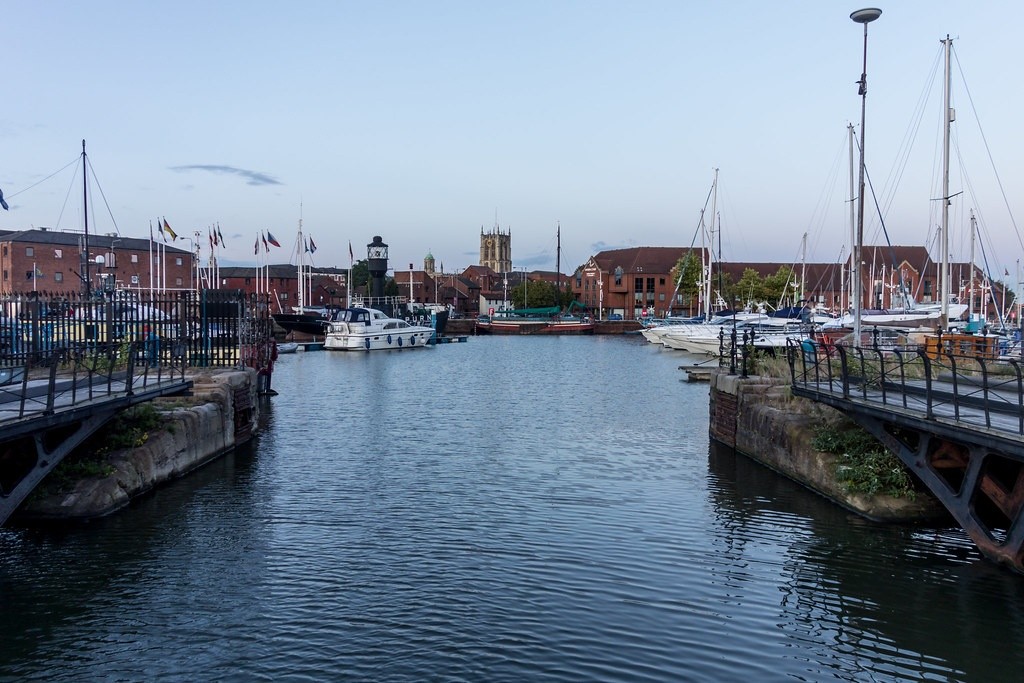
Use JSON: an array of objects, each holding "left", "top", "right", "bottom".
[
  {"left": 273, "top": 341, "right": 298, "bottom": 353},
  {"left": 322, "top": 307, "right": 435, "bottom": 350},
  {"left": 272, "top": 310, "right": 338, "bottom": 347}
]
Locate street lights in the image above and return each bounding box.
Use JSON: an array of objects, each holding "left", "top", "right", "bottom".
[
  {"left": 513, "top": 265, "right": 525, "bottom": 283},
  {"left": 849, "top": 5, "right": 883, "bottom": 355}
]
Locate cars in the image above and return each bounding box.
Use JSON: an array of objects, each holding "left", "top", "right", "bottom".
[{"left": 608, "top": 314, "right": 623, "bottom": 321}]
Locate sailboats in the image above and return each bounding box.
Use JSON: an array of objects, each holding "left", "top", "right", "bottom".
[{"left": 636, "top": 165, "right": 1023, "bottom": 360}]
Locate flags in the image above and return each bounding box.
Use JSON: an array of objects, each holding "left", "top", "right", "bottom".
[
  {"left": 209, "top": 223, "right": 225, "bottom": 251},
  {"left": 255, "top": 229, "right": 281, "bottom": 255},
  {"left": 349, "top": 242, "right": 353, "bottom": 259},
  {"left": 150, "top": 216, "right": 177, "bottom": 247},
  {"left": 304, "top": 236, "right": 317, "bottom": 254}
]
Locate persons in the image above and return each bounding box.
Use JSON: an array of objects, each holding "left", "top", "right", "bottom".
[
  {"left": 589, "top": 317, "right": 592, "bottom": 324},
  {"left": 146, "top": 330, "right": 160, "bottom": 369}
]
